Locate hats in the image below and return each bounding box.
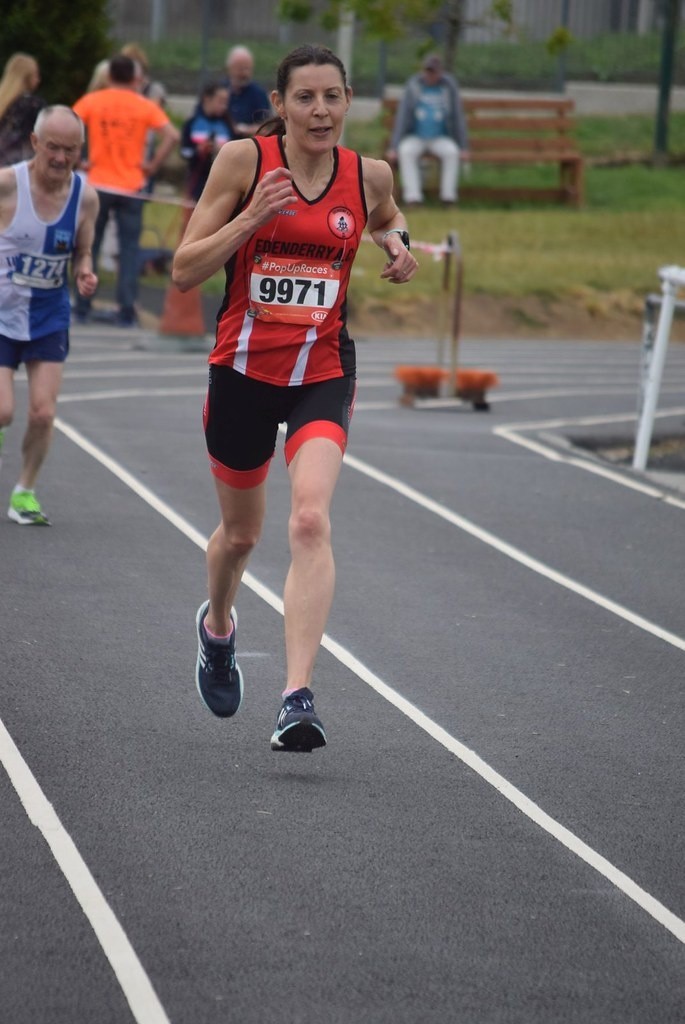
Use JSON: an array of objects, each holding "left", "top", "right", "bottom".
[{"left": 424, "top": 57, "right": 443, "bottom": 71}]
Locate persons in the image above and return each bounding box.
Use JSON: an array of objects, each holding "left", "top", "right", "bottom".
[
  {"left": 383, "top": 55, "right": 469, "bottom": 208},
  {"left": 1, "top": 103, "right": 108, "bottom": 527},
  {"left": 0, "top": 41, "right": 276, "bottom": 329},
  {"left": 172, "top": 47, "right": 422, "bottom": 753}
]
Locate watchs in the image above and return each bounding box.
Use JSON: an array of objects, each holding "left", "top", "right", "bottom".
[{"left": 382, "top": 228, "right": 411, "bottom": 251}]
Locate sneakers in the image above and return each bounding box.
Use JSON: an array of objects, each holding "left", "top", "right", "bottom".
[
  {"left": 195, "top": 596, "right": 243, "bottom": 718},
  {"left": 269, "top": 686, "right": 326, "bottom": 752},
  {"left": 8, "top": 488, "right": 49, "bottom": 526}
]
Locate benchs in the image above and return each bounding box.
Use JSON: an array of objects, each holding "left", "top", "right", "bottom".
[{"left": 378, "top": 96, "right": 583, "bottom": 206}]
